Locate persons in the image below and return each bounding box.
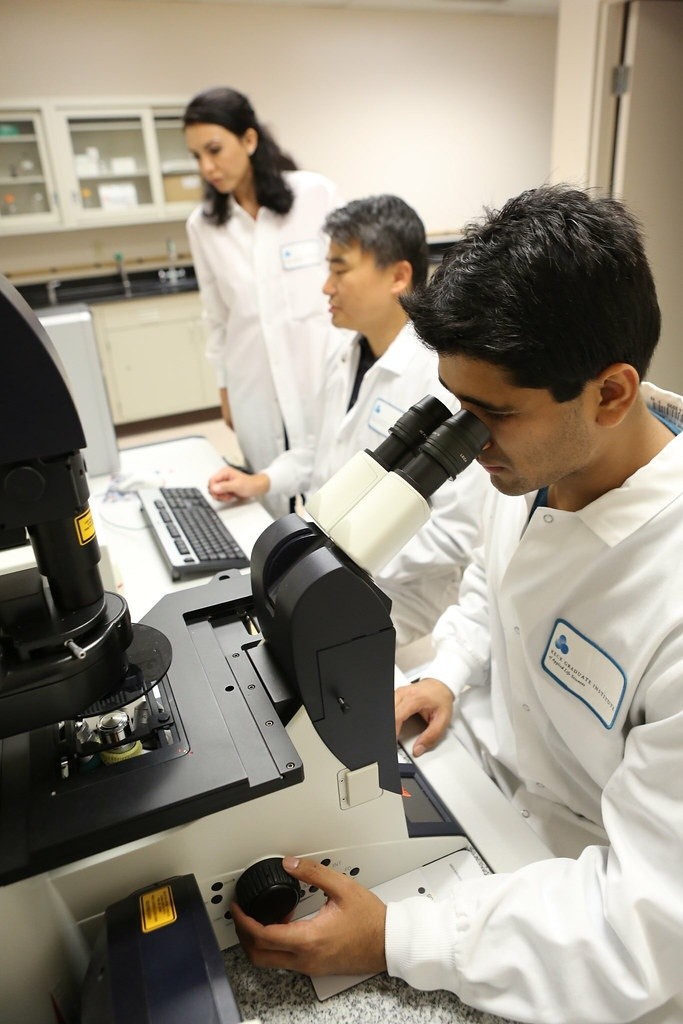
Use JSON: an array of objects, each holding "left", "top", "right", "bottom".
[
  {"left": 207, "top": 195, "right": 488, "bottom": 652},
  {"left": 184, "top": 86, "right": 349, "bottom": 519},
  {"left": 228, "top": 180, "right": 683, "bottom": 1024}
]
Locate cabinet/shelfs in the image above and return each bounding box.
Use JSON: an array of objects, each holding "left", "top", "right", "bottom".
[{"left": 0, "top": 93, "right": 222, "bottom": 424}]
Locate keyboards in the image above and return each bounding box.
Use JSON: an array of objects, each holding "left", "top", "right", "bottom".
[{"left": 136, "top": 487, "right": 250, "bottom": 582}]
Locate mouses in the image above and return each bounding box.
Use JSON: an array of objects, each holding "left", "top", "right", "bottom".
[{"left": 116, "top": 474, "right": 166, "bottom": 494}]
